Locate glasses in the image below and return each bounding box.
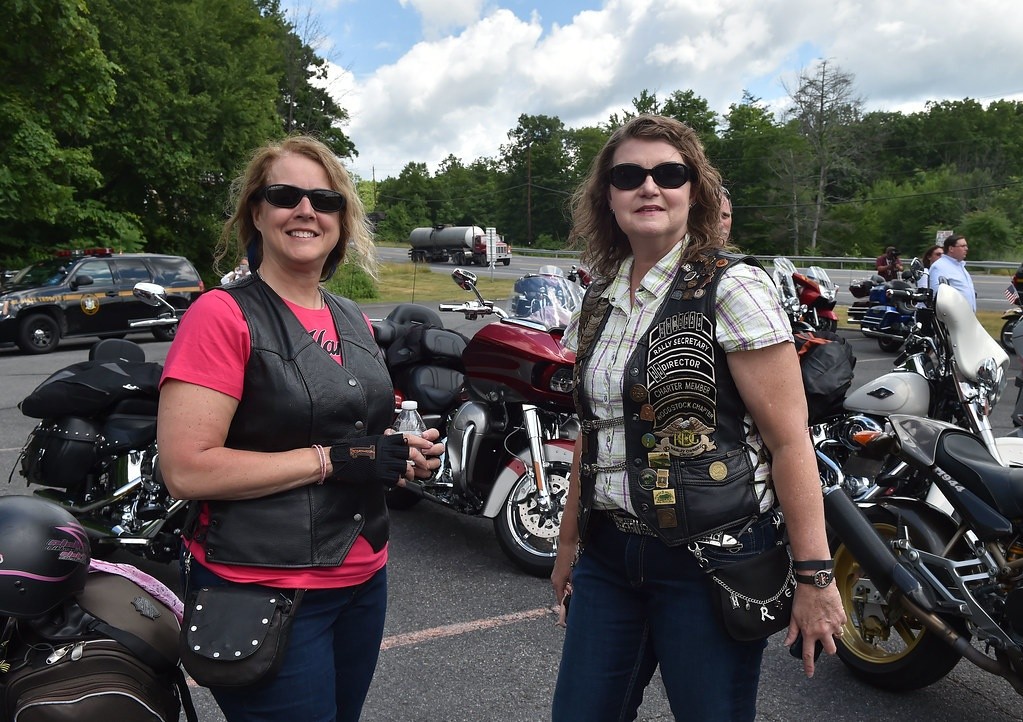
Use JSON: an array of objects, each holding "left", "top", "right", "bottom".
[
  {"left": 254, "top": 183, "right": 346, "bottom": 213},
  {"left": 935, "top": 253, "right": 944, "bottom": 256},
  {"left": 952, "top": 245, "right": 968, "bottom": 247},
  {"left": 607, "top": 162, "right": 691, "bottom": 190},
  {"left": 720, "top": 186, "right": 731, "bottom": 200}
]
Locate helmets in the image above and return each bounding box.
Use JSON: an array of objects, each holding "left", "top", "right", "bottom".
[{"left": 0, "top": 495, "right": 92, "bottom": 614}]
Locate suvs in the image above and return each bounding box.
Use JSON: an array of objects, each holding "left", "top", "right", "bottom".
[{"left": 0, "top": 247, "right": 204, "bottom": 359}]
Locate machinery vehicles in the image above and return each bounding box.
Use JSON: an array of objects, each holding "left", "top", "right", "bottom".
[{"left": 407, "top": 225, "right": 512, "bottom": 265}]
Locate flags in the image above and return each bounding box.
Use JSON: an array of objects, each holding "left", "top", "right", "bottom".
[{"left": 1005, "top": 283, "right": 1019, "bottom": 304}]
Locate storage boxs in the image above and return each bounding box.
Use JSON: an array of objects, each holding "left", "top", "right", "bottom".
[{"left": 23, "top": 414, "right": 96, "bottom": 488}]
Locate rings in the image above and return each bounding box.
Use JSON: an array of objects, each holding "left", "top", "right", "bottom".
[{"left": 833, "top": 626, "right": 845, "bottom": 638}]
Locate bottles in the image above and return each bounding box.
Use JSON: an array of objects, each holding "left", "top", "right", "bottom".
[{"left": 389, "top": 400, "right": 427, "bottom": 469}]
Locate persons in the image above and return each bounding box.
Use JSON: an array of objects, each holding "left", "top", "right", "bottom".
[
  {"left": 876, "top": 234, "right": 1023, "bottom": 313},
  {"left": 158, "top": 136, "right": 445, "bottom": 722},
  {"left": 550, "top": 115, "right": 847, "bottom": 721}
]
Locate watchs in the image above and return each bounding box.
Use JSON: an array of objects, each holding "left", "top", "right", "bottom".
[{"left": 795, "top": 570, "right": 834, "bottom": 589}]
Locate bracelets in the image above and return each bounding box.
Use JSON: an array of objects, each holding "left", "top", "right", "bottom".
[
  {"left": 792, "top": 557, "right": 834, "bottom": 571},
  {"left": 311, "top": 444, "right": 327, "bottom": 484}
]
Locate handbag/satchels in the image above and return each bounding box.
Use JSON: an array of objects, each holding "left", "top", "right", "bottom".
[
  {"left": 0, "top": 620, "right": 198, "bottom": 722},
  {"left": 386, "top": 322, "right": 472, "bottom": 374},
  {"left": 17, "top": 359, "right": 165, "bottom": 418},
  {"left": 178, "top": 584, "right": 294, "bottom": 690},
  {"left": 8, "top": 417, "right": 104, "bottom": 487},
  {"left": 789, "top": 326, "right": 858, "bottom": 421},
  {"left": 708, "top": 517, "right": 798, "bottom": 642}
]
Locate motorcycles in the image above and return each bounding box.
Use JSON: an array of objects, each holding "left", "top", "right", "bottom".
[
  {"left": 999, "top": 305, "right": 1023, "bottom": 355},
  {"left": 793, "top": 317, "right": 1023, "bottom": 692},
  {"left": 371, "top": 269, "right": 580, "bottom": 574},
  {"left": 1, "top": 334, "right": 206, "bottom": 722},
  {"left": 512, "top": 261, "right": 587, "bottom": 330},
  {"left": 846, "top": 258, "right": 940, "bottom": 352},
  {"left": 769, "top": 258, "right": 839, "bottom": 335}
]
